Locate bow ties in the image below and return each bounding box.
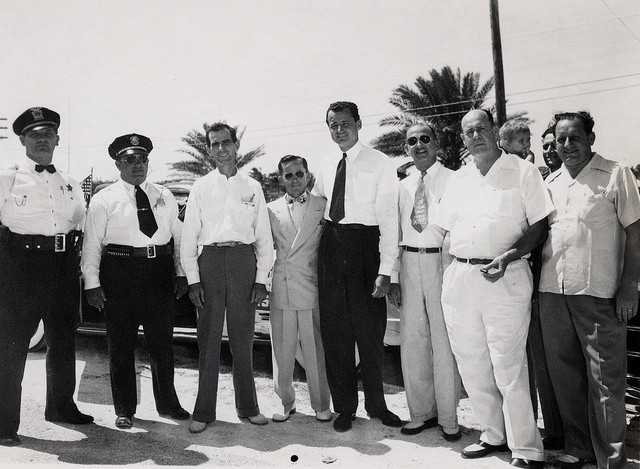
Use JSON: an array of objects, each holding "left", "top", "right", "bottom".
[
  {"left": 35, "top": 165, "right": 56, "bottom": 174},
  {"left": 287, "top": 197, "right": 306, "bottom": 204}
]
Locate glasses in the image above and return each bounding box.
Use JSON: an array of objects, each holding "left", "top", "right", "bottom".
[
  {"left": 407, "top": 135, "right": 430, "bottom": 145},
  {"left": 285, "top": 171, "right": 304, "bottom": 180},
  {"left": 119, "top": 154, "right": 148, "bottom": 164},
  {"left": 543, "top": 142, "right": 556, "bottom": 149}
]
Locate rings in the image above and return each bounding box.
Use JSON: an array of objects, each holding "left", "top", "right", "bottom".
[{"left": 628, "top": 310, "right": 632, "bottom": 312}]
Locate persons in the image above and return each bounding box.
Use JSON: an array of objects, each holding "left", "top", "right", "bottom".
[
  {"left": 79, "top": 132, "right": 191, "bottom": 429},
  {"left": 498, "top": 119, "right": 535, "bottom": 162},
  {"left": 525, "top": 126, "right": 566, "bottom": 450},
  {"left": 538, "top": 111, "right": 639, "bottom": 469},
  {"left": 386, "top": 123, "right": 466, "bottom": 443},
  {"left": 430, "top": 109, "right": 558, "bottom": 469},
  {"left": 0, "top": 107, "right": 94, "bottom": 447},
  {"left": 311, "top": 100, "right": 402, "bottom": 432},
  {"left": 179, "top": 123, "right": 275, "bottom": 434},
  {"left": 265, "top": 154, "right": 335, "bottom": 422}
]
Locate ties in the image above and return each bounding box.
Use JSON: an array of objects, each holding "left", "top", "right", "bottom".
[
  {"left": 135, "top": 185, "right": 158, "bottom": 238},
  {"left": 329, "top": 153, "right": 347, "bottom": 224},
  {"left": 411, "top": 172, "right": 428, "bottom": 234}
]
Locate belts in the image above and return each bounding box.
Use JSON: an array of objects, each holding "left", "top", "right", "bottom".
[
  {"left": 457, "top": 258, "right": 492, "bottom": 264},
  {"left": 209, "top": 241, "right": 243, "bottom": 247},
  {"left": 11, "top": 233, "right": 81, "bottom": 251},
  {"left": 331, "top": 222, "right": 364, "bottom": 229},
  {"left": 133, "top": 245, "right": 173, "bottom": 259},
  {"left": 402, "top": 246, "right": 441, "bottom": 254}
]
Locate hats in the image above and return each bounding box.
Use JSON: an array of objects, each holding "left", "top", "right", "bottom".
[
  {"left": 12, "top": 107, "right": 60, "bottom": 135},
  {"left": 108, "top": 134, "right": 153, "bottom": 160}
]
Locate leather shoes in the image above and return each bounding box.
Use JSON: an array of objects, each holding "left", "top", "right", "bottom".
[
  {"left": 189, "top": 420, "right": 207, "bottom": 433},
  {"left": 334, "top": 412, "right": 356, "bottom": 432},
  {"left": 45, "top": 409, "right": 94, "bottom": 424},
  {"left": 1, "top": 432, "right": 20, "bottom": 447},
  {"left": 315, "top": 409, "right": 332, "bottom": 422},
  {"left": 401, "top": 417, "right": 437, "bottom": 435},
  {"left": 272, "top": 404, "right": 296, "bottom": 422},
  {"left": 556, "top": 453, "right": 597, "bottom": 469},
  {"left": 158, "top": 407, "right": 190, "bottom": 419},
  {"left": 543, "top": 437, "right": 565, "bottom": 450},
  {"left": 368, "top": 409, "right": 402, "bottom": 427},
  {"left": 442, "top": 426, "right": 461, "bottom": 441},
  {"left": 510, "top": 458, "right": 532, "bottom": 469},
  {"left": 238, "top": 413, "right": 268, "bottom": 425},
  {"left": 462, "top": 439, "right": 506, "bottom": 457},
  {"left": 115, "top": 416, "right": 134, "bottom": 428}
]
